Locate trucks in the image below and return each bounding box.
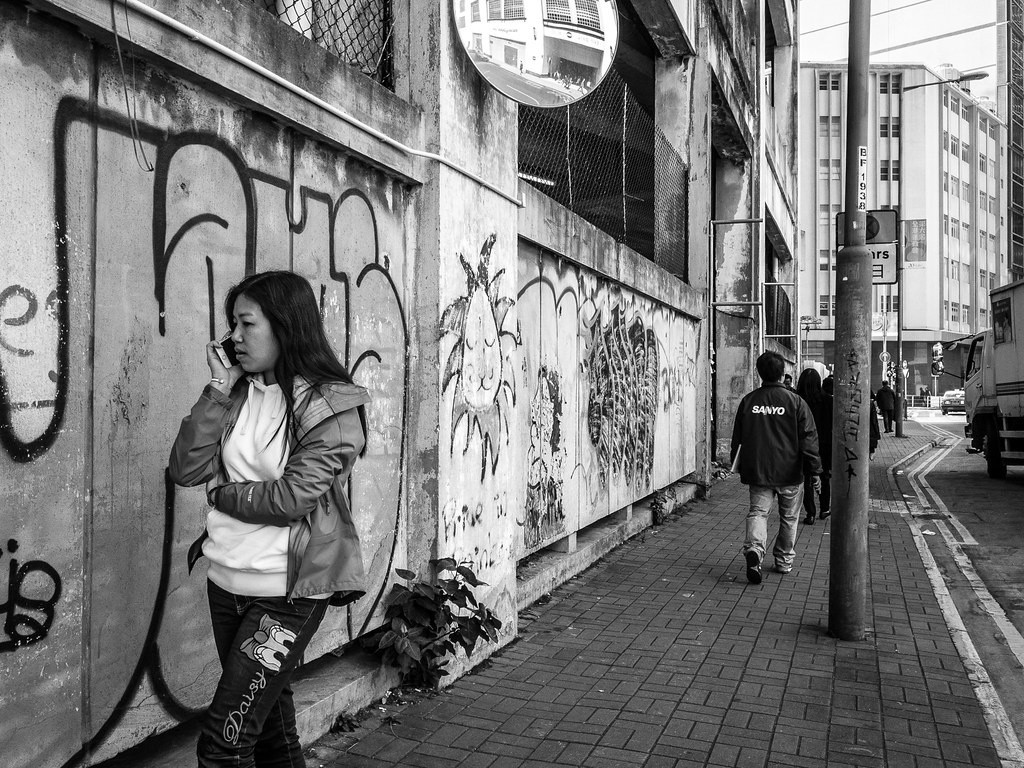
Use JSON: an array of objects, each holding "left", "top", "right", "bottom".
[{"left": 930, "top": 278, "right": 1024, "bottom": 480}]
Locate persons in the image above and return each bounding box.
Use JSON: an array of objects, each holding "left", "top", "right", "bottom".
[
  {"left": 730, "top": 351, "right": 821, "bottom": 585},
  {"left": 823, "top": 374, "right": 833, "bottom": 397},
  {"left": 876, "top": 380, "right": 896, "bottom": 433},
  {"left": 520, "top": 61, "right": 523, "bottom": 73},
  {"left": 784, "top": 374, "right": 797, "bottom": 394},
  {"left": 920, "top": 387, "right": 931, "bottom": 407},
  {"left": 869, "top": 386, "right": 881, "bottom": 461},
  {"left": 796, "top": 368, "right": 833, "bottom": 525},
  {"left": 166, "top": 270, "right": 371, "bottom": 768}
]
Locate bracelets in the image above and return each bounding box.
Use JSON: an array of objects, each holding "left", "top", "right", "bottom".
[
  {"left": 211, "top": 378, "right": 234, "bottom": 391},
  {"left": 206, "top": 487, "right": 216, "bottom": 507}
]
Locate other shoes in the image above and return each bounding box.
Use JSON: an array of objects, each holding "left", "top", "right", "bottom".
[
  {"left": 747, "top": 547, "right": 762, "bottom": 585},
  {"left": 803, "top": 517, "right": 815, "bottom": 525},
  {"left": 776, "top": 566, "right": 793, "bottom": 574},
  {"left": 820, "top": 511, "right": 831, "bottom": 519},
  {"left": 884, "top": 429, "right": 889, "bottom": 433},
  {"left": 889, "top": 429, "right": 893, "bottom": 432}
]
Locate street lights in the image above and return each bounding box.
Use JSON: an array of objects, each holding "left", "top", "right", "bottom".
[{"left": 893, "top": 68, "right": 992, "bottom": 437}]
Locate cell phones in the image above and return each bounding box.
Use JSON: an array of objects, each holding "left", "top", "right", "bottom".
[
  {"left": 811, "top": 477, "right": 819, "bottom": 496},
  {"left": 214, "top": 335, "right": 241, "bottom": 369}
]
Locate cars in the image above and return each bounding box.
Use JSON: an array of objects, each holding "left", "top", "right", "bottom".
[{"left": 941, "top": 389, "right": 965, "bottom": 415}]
